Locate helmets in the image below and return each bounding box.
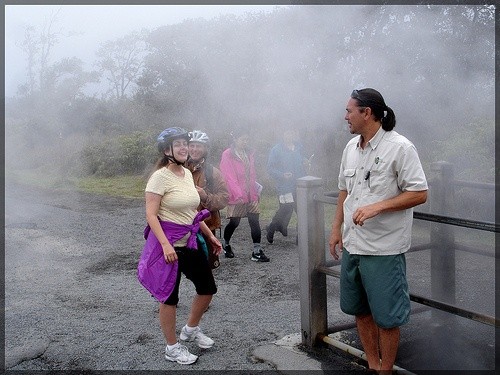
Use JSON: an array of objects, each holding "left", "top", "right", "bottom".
[
  {"left": 157, "top": 126, "right": 188, "bottom": 144},
  {"left": 187, "top": 129, "right": 209, "bottom": 144}
]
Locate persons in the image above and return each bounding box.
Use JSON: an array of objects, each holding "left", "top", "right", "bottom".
[
  {"left": 266, "top": 125, "right": 306, "bottom": 246},
  {"left": 221, "top": 128, "right": 270, "bottom": 262},
  {"left": 181, "top": 130, "right": 228, "bottom": 311},
  {"left": 330, "top": 87, "right": 428, "bottom": 375},
  {"left": 145, "top": 126, "right": 224, "bottom": 365}
]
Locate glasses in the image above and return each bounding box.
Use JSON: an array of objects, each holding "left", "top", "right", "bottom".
[{"left": 351, "top": 90, "right": 367, "bottom": 105}]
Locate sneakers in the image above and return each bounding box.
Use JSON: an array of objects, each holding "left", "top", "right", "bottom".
[
  {"left": 179, "top": 324, "right": 215, "bottom": 349},
  {"left": 164, "top": 342, "right": 199, "bottom": 365}
]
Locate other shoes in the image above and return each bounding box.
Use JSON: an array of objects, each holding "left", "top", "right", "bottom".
[
  {"left": 222, "top": 243, "right": 234, "bottom": 258},
  {"left": 251, "top": 249, "right": 270, "bottom": 262},
  {"left": 265, "top": 224, "right": 275, "bottom": 244}
]
